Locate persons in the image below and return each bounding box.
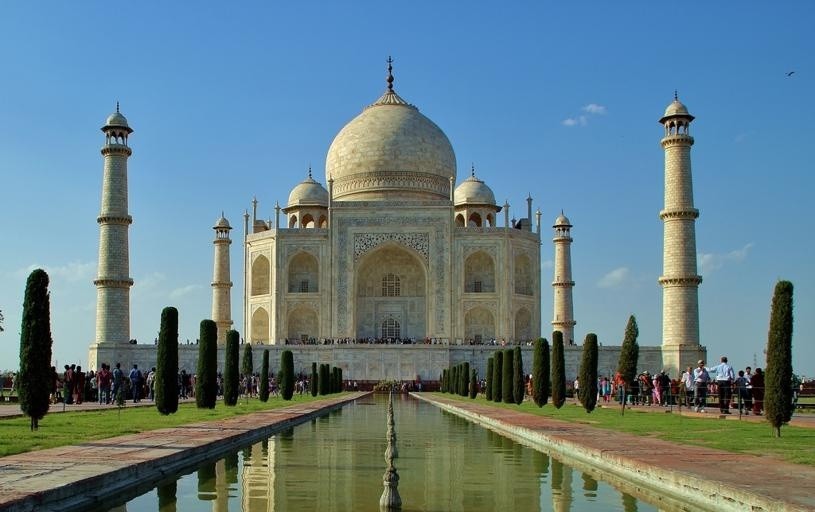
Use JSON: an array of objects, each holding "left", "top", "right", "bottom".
[
  {"left": 180, "top": 338, "right": 199, "bottom": 346},
  {"left": 344, "top": 380, "right": 358, "bottom": 392},
  {"left": 269, "top": 377, "right": 280, "bottom": 399},
  {"left": 178, "top": 367, "right": 197, "bottom": 400},
  {"left": 155, "top": 338, "right": 157, "bottom": 345},
  {"left": 217, "top": 374, "right": 224, "bottom": 400},
  {"left": 240, "top": 374, "right": 260, "bottom": 399},
  {"left": 393, "top": 379, "right": 422, "bottom": 393},
  {"left": 294, "top": 376, "right": 311, "bottom": 396},
  {"left": 478, "top": 356, "right": 767, "bottom": 415},
  {"left": 284, "top": 334, "right": 535, "bottom": 347},
  {"left": 9, "top": 372, "right": 17, "bottom": 395},
  {"left": 49, "top": 362, "right": 156, "bottom": 406},
  {"left": 128, "top": 338, "right": 138, "bottom": 343},
  {"left": 241, "top": 337, "right": 243, "bottom": 346}
]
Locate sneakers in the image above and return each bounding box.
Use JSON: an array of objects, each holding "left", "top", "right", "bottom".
[{"left": 695, "top": 406, "right": 704, "bottom": 413}]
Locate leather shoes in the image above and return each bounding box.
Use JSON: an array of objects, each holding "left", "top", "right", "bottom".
[{"left": 721, "top": 410, "right": 731, "bottom": 414}]
